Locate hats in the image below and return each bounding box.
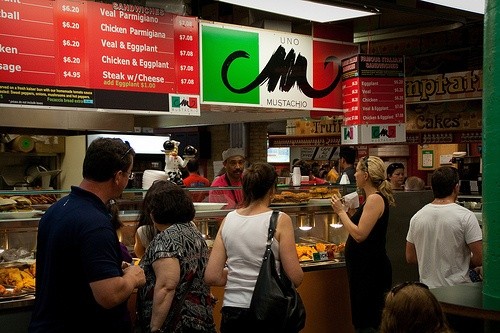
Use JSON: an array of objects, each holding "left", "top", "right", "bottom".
[
  {"left": 222, "top": 147, "right": 245, "bottom": 162},
  {"left": 119, "top": 140, "right": 135, "bottom": 161}
]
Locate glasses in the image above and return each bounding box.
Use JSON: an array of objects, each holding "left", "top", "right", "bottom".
[
  {"left": 391, "top": 281, "right": 430, "bottom": 296},
  {"left": 115, "top": 170, "right": 135, "bottom": 180}
]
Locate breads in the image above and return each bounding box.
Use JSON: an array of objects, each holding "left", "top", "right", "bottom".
[
  {"left": 271, "top": 186, "right": 341, "bottom": 203},
  {"left": 296, "top": 242, "right": 327, "bottom": 260}
]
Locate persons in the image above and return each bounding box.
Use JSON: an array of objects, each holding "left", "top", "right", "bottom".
[
  {"left": 133, "top": 179, "right": 198, "bottom": 258},
  {"left": 385, "top": 162, "right": 405, "bottom": 191},
  {"left": 328, "top": 148, "right": 360, "bottom": 185},
  {"left": 135, "top": 178, "right": 217, "bottom": 333},
  {"left": 182, "top": 160, "right": 212, "bottom": 203},
  {"left": 205, "top": 161, "right": 304, "bottom": 333},
  {"left": 332, "top": 156, "right": 392, "bottom": 333},
  {"left": 36, "top": 137, "right": 145, "bottom": 333},
  {"left": 379, "top": 280, "right": 449, "bottom": 333},
  {"left": 206, "top": 149, "right": 251, "bottom": 210},
  {"left": 406, "top": 165, "right": 482, "bottom": 290}
]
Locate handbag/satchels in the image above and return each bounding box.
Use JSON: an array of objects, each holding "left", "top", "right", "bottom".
[{"left": 248, "top": 209, "right": 306, "bottom": 333}]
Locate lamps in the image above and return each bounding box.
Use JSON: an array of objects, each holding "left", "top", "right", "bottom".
[
  {"left": 299, "top": 215, "right": 313, "bottom": 230},
  {"left": 330, "top": 214, "right": 344, "bottom": 229}
]
